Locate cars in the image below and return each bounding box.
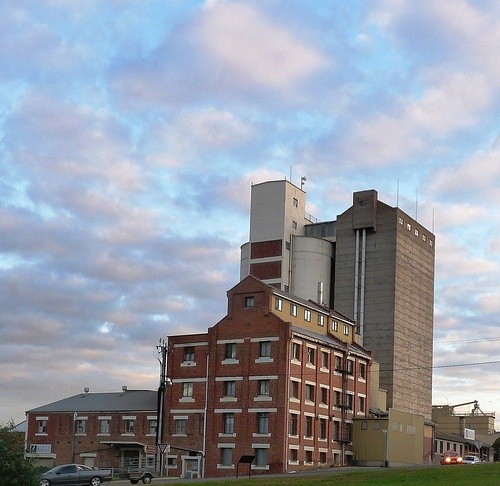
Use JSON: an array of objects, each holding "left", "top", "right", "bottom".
[{"left": 439, "top": 451, "right": 479, "bottom": 466}]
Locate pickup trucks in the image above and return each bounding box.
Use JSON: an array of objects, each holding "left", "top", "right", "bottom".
[{"left": 36, "top": 463, "right": 112, "bottom": 486}]
